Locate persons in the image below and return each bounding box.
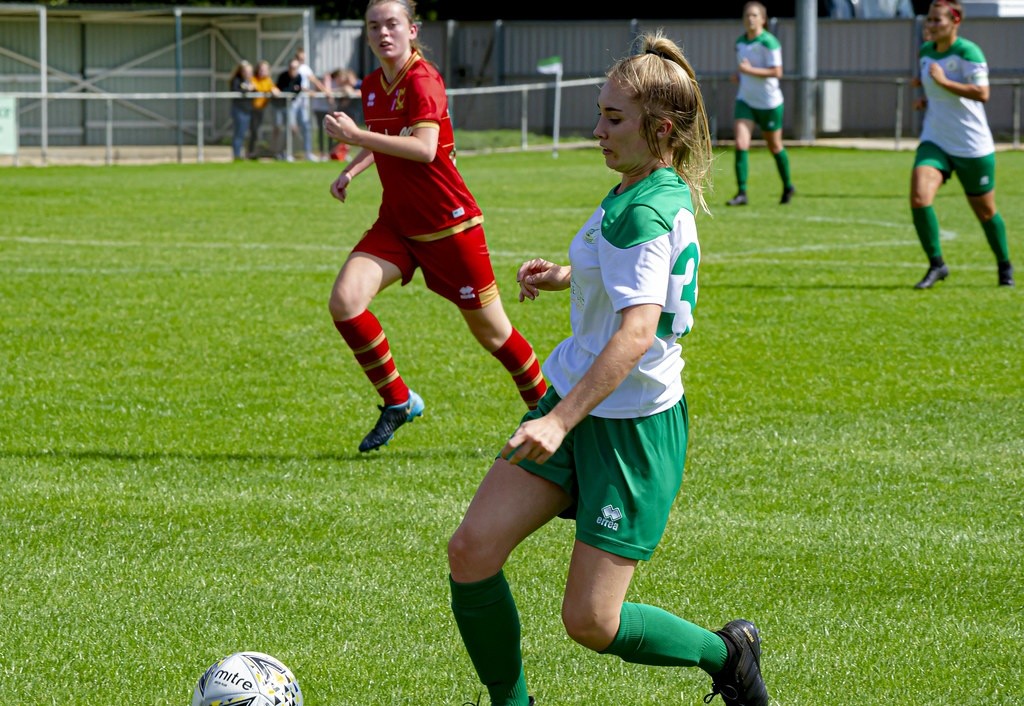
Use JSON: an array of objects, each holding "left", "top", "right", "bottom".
[
  {"left": 250, "top": 60, "right": 281, "bottom": 160},
  {"left": 273, "top": 60, "right": 303, "bottom": 162},
  {"left": 327, "top": 1, "right": 548, "bottom": 455},
  {"left": 909, "top": 0, "right": 1017, "bottom": 290},
  {"left": 312, "top": 69, "right": 367, "bottom": 162},
  {"left": 230, "top": 59, "right": 254, "bottom": 161},
  {"left": 439, "top": 32, "right": 779, "bottom": 706},
  {"left": 290, "top": 47, "right": 330, "bottom": 164},
  {"left": 725, "top": 2, "right": 798, "bottom": 207}
]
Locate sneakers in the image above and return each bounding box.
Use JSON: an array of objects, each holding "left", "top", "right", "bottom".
[
  {"left": 715, "top": 619, "right": 769, "bottom": 706},
  {"left": 998, "top": 262, "right": 1013, "bottom": 285},
  {"left": 913, "top": 264, "right": 948, "bottom": 289},
  {"left": 358, "top": 390, "right": 424, "bottom": 453},
  {"left": 726, "top": 194, "right": 747, "bottom": 206},
  {"left": 779, "top": 186, "right": 794, "bottom": 203}
]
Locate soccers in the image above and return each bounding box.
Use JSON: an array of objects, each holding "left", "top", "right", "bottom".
[{"left": 193, "top": 652, "right": 302, "bottom": 706}]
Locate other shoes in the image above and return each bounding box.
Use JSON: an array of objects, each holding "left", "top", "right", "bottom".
[
  {"left": 305, "top": 153, "right": 318, "bottom": 162},
  {"left": 321, "top": 152, "right": 330, "bottom": 162},
  {"left": 345, "top": 154, "right": 352, "bottom": 161},
  {"left": 246, "top": 153, "right": 258, "bottom": 161},
  {"left": 287, "top": 156, "right": 297, "bottom": 163}
]
